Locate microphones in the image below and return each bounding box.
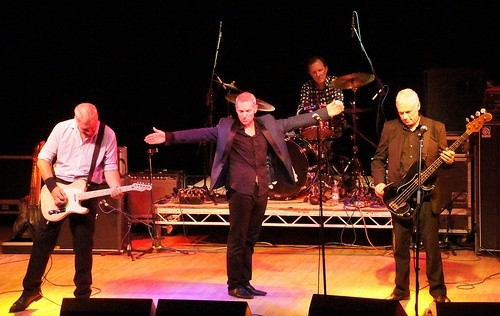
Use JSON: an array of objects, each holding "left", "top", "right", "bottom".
[
  {"left": 215, "top": 74, "right": 227, "bottom": 90},
  {"left": 98, "top": 199, "right": 106, "bottom": 205},
  {"left": 418, "top": 126, "right": 428, "bottom": 137},
  {"left": 352, "top": 15, "right": 355, "bottom": 38},
  {"left": 372, "top": 89, "right": 382, "bottom": 100}
]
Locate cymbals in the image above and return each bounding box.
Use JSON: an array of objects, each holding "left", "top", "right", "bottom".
[
  {"left": 213, "top": 79, "right": 244, "bottom": 92},
  {"left": 225, "top": 93, "right": 276, "bottom": 112},
  {"left": 330, "top": 70, "right": 376, "bottom": 90},
  {"left": 338, "top": 105, "right": 374, "bottom": 114}
]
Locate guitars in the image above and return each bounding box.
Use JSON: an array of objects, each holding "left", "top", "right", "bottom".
[
  {"left": 12, "top": 141, "right": 46, "bottom": 239},
  {"left": 381, "top": 107, "right": 493, "bottom": 220},
  {"left": 40, "top": 178, "right": 154, "bottom": 223}
]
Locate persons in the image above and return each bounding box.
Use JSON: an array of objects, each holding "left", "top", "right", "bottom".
[
  {"left": 370, "top": 88, "right": 455, "bottom": 303},
  {"left": 9, "top": 103, "right": 125, "bottom": 312},
  {"left": 144, "top": 91, "right": 344, "bottom": 298},
  {"left": 293, "top": 57, "right": 357, "bottom": 193}
]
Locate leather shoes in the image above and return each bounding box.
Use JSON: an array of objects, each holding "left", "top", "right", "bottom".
[
  {"left": 244, "top": 285, "right": 267, "bottom": 296},
  {"left": 385, "top": 293, "right": 410, "bottom": 301},
  {"left": 8, "top": 290, "right": 43, "bottom": 313},
  {"left": 229, "top": 286, "right": 254, "bottom": 299},
  {"left": 433, "top": 295, "right": 451, "bottom": 303}
]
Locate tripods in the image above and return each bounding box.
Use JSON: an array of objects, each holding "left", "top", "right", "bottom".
[
  {"left": 132, "top": 148, "right": 188, "bottom": 261},
  {"left": 305, "top": 110, "right": 372, "bottom": 194}
]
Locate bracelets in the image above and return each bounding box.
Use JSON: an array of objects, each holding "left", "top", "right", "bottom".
[{"left": 44, "top": 177, "right": 58, "bottom": 192}]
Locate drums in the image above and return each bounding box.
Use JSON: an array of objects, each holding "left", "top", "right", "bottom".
[
  {"left": 266, "top": 133, "right": 309, "bottom": 201},
  {"left": 297, "top": 105, "right": 336, "bottom": 141}
]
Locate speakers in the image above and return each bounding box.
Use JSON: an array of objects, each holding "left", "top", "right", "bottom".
[
  {"left": 127, "top": 169, "right": 184, "bottom": 215},
  {"left": 474, "top": 122, "right": 500, "bottom": 251},
  {"left": 421, "top": 301, "right": 500, "bottom": 316},
  {"left": 308, "top": 294, "right": 409, "bottom": 316},
  {"left": 52, "top": 177, "right": 127, "bottom": 256},
  {"left": 156, "top": 298, "right": 253, "bottom": 316},
  {"left": 60, "top": 298, "right": 156, "bottom": 316}
]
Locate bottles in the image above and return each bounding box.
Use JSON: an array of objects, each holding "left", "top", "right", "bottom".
[{"left": 332, "top": 184, "right": 339, "bottom": 205}]
